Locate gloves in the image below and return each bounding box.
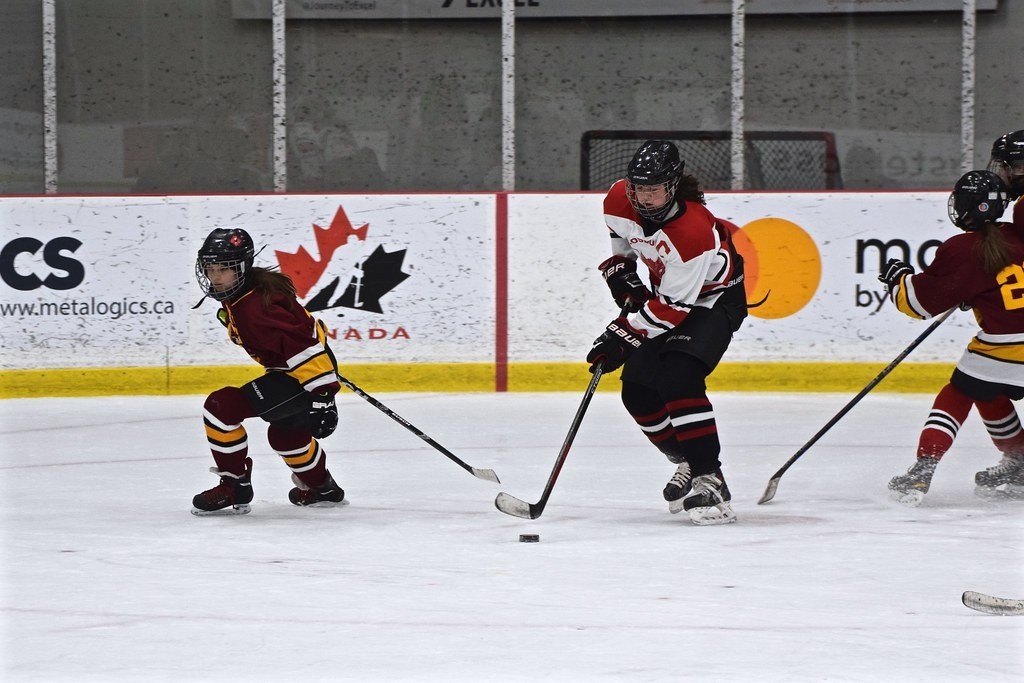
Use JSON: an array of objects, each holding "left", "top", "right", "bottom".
[
  {"left": 308, "top": 390, "right": 338, "bottom": 439},
  {"left": 587, "top": 317, "right": 649, "bottom": 374},
  {"left": 598, "top": 255, "right": 652, "bottom": 313},
  {"left": 878, "top": 258, "right": 914, "bottom": 285}
]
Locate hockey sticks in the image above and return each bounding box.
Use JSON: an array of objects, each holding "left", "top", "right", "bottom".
[
  {"left": 960, "top": 590, "right": 1023, "bottom": 618},
  {"left": 338, "top": 376, "right": 501, "bottom": 484},
  {"left": 495, "top": 364, "right": 606, "bottom": 519},
  {"left": 759, "top": 303, "right": 959, "bottom": 502}
]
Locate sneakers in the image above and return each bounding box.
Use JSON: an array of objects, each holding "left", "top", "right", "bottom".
[
  {"left": 683, "top": 468, "right": 738, "bottom": 526},
  {"left": 888, "top": 458, "right": 938, "bottom": 507},
  {"left": 975, "top": 453, "right": 1024, "bottom": 501},
  {"left": 663, "top": 460, "right": 693, "bottom": 514},
  {"left": 288, "top": 469, "right": 350, "bottom": 508},
  {"left": 190, "top": 457, "right": 254, "bottom": 516}
]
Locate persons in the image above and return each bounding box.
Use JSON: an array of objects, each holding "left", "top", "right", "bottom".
[
  {"left": 878, "top": 129, "right": 1024, "bottom": 505},
  {"left": 192, "top": 228, "right": 344, "bottom": 514},
  {"left": 587, "top": 139, "right": 749, "bottom": 525}
]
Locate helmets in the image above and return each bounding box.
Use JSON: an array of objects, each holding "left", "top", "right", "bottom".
[
  {"left": 627, "top": 140, "right": 686, "bottom": 221},
  {"left": 991, "top": 130, "right": 1024, "bottom": 195},
  {"left": 198, "top": 228, "right": 255, "bottom": 303},
  {"left": 954, "top": 170, "right": 1010, "bottom": 226}
]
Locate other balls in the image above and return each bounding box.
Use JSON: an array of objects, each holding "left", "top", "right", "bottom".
[{"left": 517, "top": 530, "right": 542, "bottom": 544}]
